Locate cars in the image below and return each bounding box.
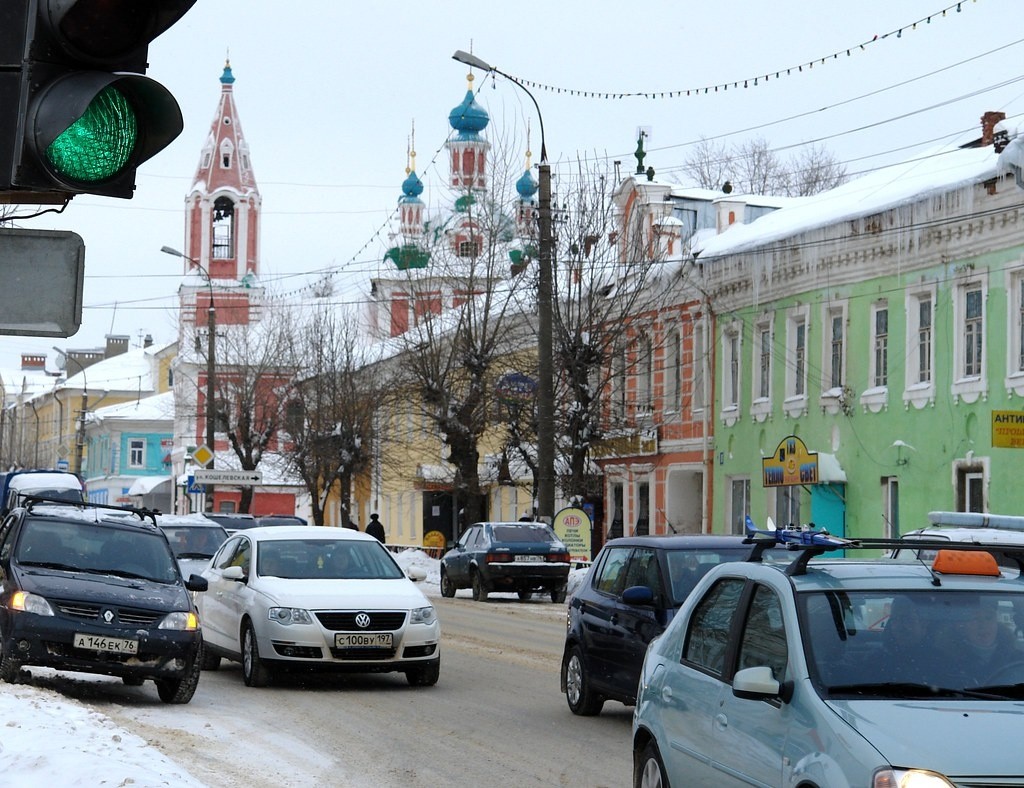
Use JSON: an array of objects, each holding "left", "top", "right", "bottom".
[
  {"left": 192, "top": 526, "right": 440, "bottom": 688},
  {"left": 0, "top": 493, "right": 208, "bottom": 707},
  {"left": 633, "top": 516, "right": 1023, "bottom": 787},
  {"left": 440, "top": 522, "right": 571, "bottom": 603},
  {"left": 145, "top": 515, "right": 230, "bottom": 597},
  {"left": 561, "top": 535, "right": 805, "bottom": 717},
  {"left": 859, "top": 511, "right": 1023, "bottom": 631}
]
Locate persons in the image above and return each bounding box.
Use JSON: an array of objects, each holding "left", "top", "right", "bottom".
[
  {"left": 949, "top": 601, "right": 1018, "bottom": 674},
  {"left": 192, "top": 534, "right": 213, "bottom": 552},
  {"left": 670, "top": 553, "right": 698, "bottom": 590},
  {"left": 332, "top": 550, "right": 359, "bottom": 575},
  {"left": 365, "top": 513, "right": 385, "bottom": 543}
]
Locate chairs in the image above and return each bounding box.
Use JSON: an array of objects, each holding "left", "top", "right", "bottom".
[
  {"left": 809, "top": 613, "right": 863, "bottom": 686},
  {"left": 100, "top": 542, "right": 136, "bottom": 564},
  {"left": 25, "top": 531, "right": 65, "bottom": 563}
]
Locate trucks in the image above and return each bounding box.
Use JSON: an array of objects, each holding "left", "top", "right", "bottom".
[{"left": 0, "top": 469, "right": 86, "bottom": 524}]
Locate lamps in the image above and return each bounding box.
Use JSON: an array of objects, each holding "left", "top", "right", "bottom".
[{"left": 888, "top": 439, "right": 914, "bottom": 465}]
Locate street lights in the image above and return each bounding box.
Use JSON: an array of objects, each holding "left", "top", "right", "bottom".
[
  {"left": 161, "top": 246, "right": 216, "bottom": 513},
  {"left": 53, "top": 346, "right": 87, "bottom": 474},
  {"left": 453, "top": 49, "right": 555, "bottom": 529}
]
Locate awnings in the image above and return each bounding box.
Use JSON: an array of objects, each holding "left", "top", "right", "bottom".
[{"left": 415, "top": 463, "right": 492, "bottom": 490}]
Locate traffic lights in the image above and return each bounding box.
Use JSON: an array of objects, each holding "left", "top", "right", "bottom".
[{"left": 12, "top": 1, "right": 197, "bottom": 199}]
